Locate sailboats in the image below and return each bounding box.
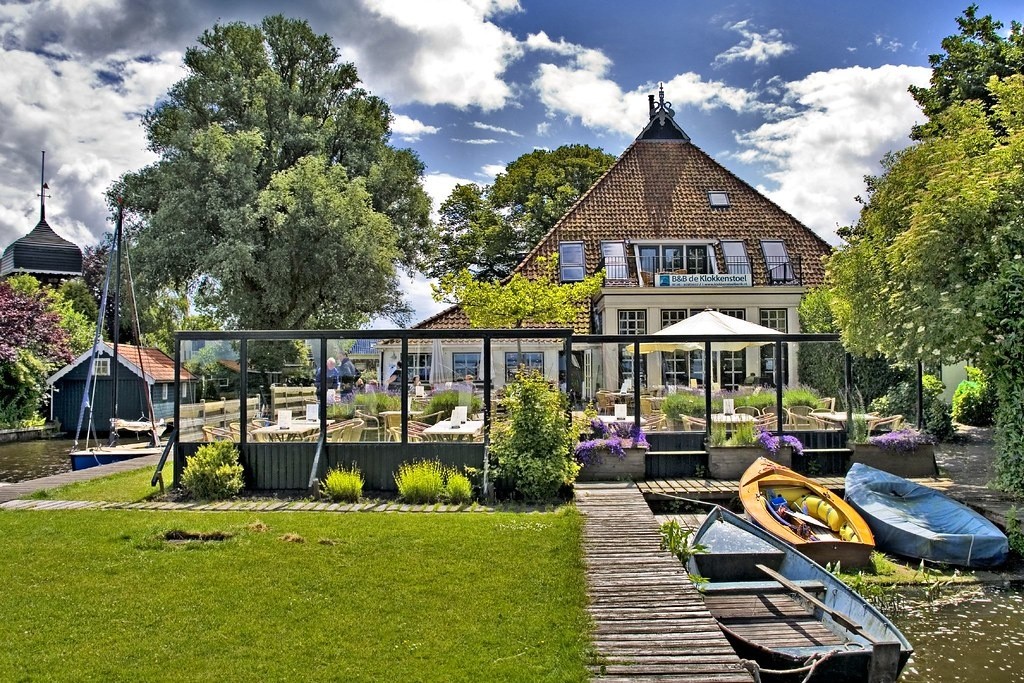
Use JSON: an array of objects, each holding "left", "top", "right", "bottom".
[{"left": 65, "top": 203, "right": 175, "bottom": 472}]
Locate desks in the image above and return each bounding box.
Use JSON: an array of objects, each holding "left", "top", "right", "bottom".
[
  {"left": 251, "top": 418, "right": 335, "bottom": 441},
  {"left": 598, "top": 415, "right": 648, "bottom": 425},
  {"left": 704, "top": 413, "right": 759, "bottom": 428},
  {"left": 613, "top": 391, "right": 633, "bottom": 404},
  {"left": 424, "top": 421, "right": 484, "bottom": 441},
  {"left": 812, "top": 411, "right": 879, "bottom": 433}
]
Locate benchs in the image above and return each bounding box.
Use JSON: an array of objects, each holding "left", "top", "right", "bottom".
[{"left": 704, "top": 578, "right": 823, "bottom": 599}]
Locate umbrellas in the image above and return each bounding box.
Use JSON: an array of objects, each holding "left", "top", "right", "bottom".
[{"left": 625, "top": 308, "right": 792, "bottom": 392}]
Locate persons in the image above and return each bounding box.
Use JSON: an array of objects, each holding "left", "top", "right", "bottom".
[
  {"left": 744, "top": 373, "right": 757, "bottom": 385},
  {"left": 315, "top": 357, "right": 338, "bottom": 404},
  {"left": 386, "top": 361, "right": 401, "bottom": 392},
  {"left": 412, "top": 374, "right": 422, "bottom": 386},
  {"left": 337, "top": 351, "right": 361, "bottom": 402},
  {"left": 450, "top": 375, "right": 476, "bottom": 394}
]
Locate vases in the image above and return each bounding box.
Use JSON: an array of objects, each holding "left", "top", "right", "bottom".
[
  {"left": 846, "top": 442, "right": 937, "bottom": 476},
  {"left": 703, "top": 443, "right": 796, "bottom": 476},
  {"left": 578, "top": 446, "right": 649, "bottom": 480}
]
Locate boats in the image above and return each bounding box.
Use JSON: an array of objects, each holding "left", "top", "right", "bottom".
[
  {"left": 842, "top": 460, "right": 1010, "bottom": 568},
  {"left": 686, "top": 503, "right": 915, "bottom": 683},
  {"left": 738, "top": 454, "right": 876, "bottom": 574}
]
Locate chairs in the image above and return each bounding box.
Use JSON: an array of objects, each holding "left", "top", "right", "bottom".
[
  {"left": 640, "top": 269, "right": 688, "bottom": 287},
  {"left": 201, "top": 378, "right": 900, "bottom": 442}
]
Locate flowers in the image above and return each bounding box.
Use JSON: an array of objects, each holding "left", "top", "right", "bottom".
[
  {"left": 846, "top": 412, "right": 939, "bottom": 453},
  {"left": 708, "top": 420, "right": 804, "bottom": 457},
  {"left": 578, "top": 419, "right": 653, "bottom": 467}
]
